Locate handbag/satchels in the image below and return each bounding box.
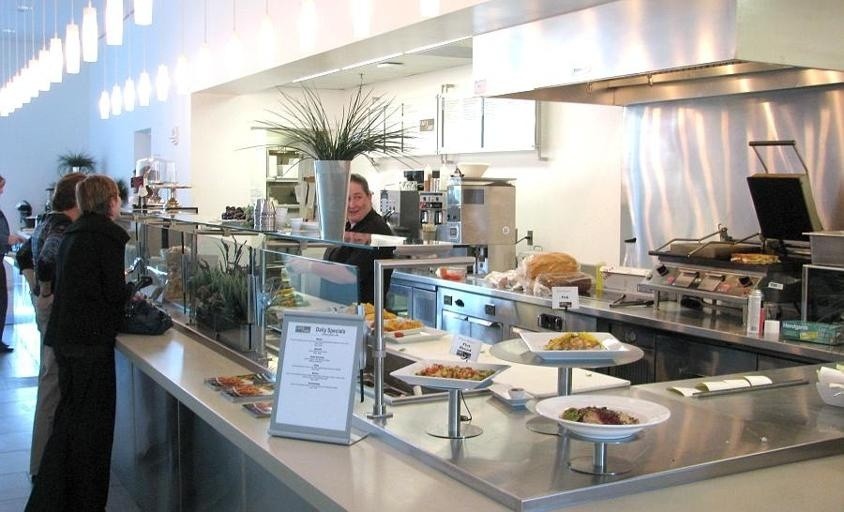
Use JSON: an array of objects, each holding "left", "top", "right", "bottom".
[{"left": 119, "top": 298, "right": 174, "bottom": 336}]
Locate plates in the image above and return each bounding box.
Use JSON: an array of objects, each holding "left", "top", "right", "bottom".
[
  {"left": 388, "top": 356, "right": 513, "bottom": 389},
  {"left": 488, "top": 385, "right": 534, "bottom": 408},
  {"left": 217, "top": 218, "right": 244, "bottom": 223},
  {"left": 520, "top": 327, "right": 631, "bottom": 362},
  {"left": 347, "top": 308, "right": 449, "bottom": 343},
  {"left": 534, "top": 393, "right": 671, "bottom": 440}
]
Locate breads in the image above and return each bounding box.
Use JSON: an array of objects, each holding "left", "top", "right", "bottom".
[{"left": 487, "top": 250, "right": 590, "bottom": 298}]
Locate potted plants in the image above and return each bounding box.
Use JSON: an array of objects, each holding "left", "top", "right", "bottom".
[
  {"left": 234, "top": 67, "right": 421, "bottom": 242},
  {"left": 56, "top": 146, "right": 95, "bottom": 177},
  {"left": 185, "top": 240, "right": 278, "bottom": 332}
]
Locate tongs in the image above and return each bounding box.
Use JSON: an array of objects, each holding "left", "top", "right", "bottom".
[{"left": 608, "top": 291, "right": 653, "bottom": 308}]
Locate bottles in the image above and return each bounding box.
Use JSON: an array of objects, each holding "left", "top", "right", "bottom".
[
  {"left": 432, "top": 170, "right": 441, "bottom": 192},
  {"left": 746, "top": 288, "right": 762, "bottom": 334}
]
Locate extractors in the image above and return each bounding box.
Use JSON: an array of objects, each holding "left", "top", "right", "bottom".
[{"left": 472, "top": 0, "right": 844, "bottom": 107}]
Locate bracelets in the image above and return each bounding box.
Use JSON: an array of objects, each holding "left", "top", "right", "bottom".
[
  {"left": 308, "top": 262, "right": 312, "bottom": 274},
  {"left": 42, "top": 293, "right": 50, "bottom": 297}
]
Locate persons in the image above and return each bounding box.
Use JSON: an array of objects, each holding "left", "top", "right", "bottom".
[
  {"left": 15, "top": 173, "right": 86, "bottom": 485},
  {"left": 25, "top": 175, "right": 138, "bottom": 511},
  {"left": 0, "top": 175, "right": 22, "bottom": 352},
  {"left": 287, "top": 174, "right": 396, "bottom": 309}
]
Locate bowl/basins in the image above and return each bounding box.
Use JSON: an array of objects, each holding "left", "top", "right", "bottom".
[
  {"left": 439, "top": 267, "right": 467, "bottom": 280},
  {"left": 507, "top": 388, "right": 525, "bottom": 400},
  {"left": 455, "top": 163, "right": 488, "bottom": 179}
]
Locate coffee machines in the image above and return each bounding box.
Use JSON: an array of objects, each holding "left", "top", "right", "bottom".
[{"left": 418, "top": 190, "right": 447, "bottom": 226}]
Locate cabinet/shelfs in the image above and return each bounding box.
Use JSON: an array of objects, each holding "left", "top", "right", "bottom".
[
  {"left": 443, "top": 88, "right": 542, "bottom": 161},
  {"left": 401, "top": 95, "right": 437, "bottom": 157},
  {"left": 370, "top": 97, "right": 402, "bottom": 156}
]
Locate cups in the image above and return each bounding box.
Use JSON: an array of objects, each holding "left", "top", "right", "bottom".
[
  {"left": 276, "top": 207, "right": 288, "bottom": 230},
  {"left": 764, "top": 319, "right": 781, "bottom": 334},
  {"left": 253, "top": 198, "right": 276, "bottom": 233},
  {"left": 398, "top": 180, "right": 418, "bottom": 191},
  {"left": 290, "top": 217, "right": 303, "bottom": 232}
]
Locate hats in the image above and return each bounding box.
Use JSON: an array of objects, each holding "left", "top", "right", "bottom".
[{"left": 135, "top": 158, "right": 150, "bottom": 178}]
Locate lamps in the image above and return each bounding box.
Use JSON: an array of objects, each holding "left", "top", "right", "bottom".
[{"left": 0, "top": 0, "right": 171, "bottom": 119}]
